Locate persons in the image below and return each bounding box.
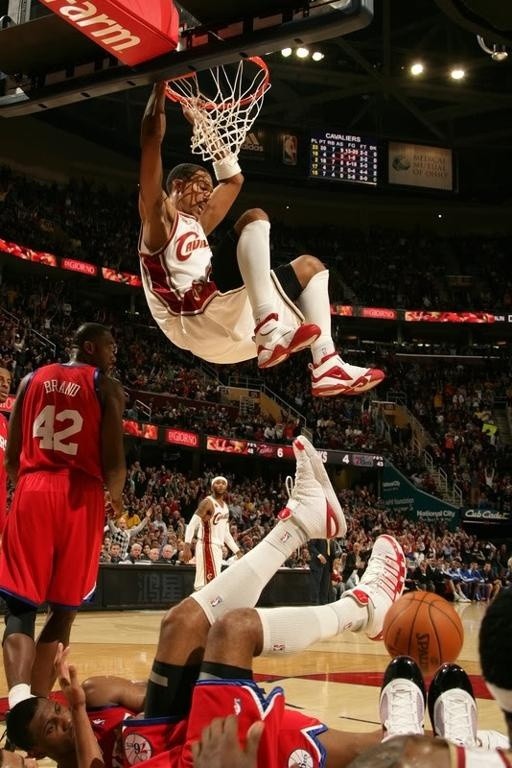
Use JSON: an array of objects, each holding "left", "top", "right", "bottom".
[
  {"left": 1, "top": 436, "right": 512, "bottom": 768},
  {"left": 138, "top": 81, "right": 384, "bottom": 396},
  {"left": 2, "top": 322, "right": 126, "bottom": 712},
  {"left": 1, "top": 162, "right": 512, "bottom": 460}
]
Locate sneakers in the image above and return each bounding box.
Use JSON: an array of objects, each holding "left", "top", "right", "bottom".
[
  {"left": 379, "top": 656, "right": 428, "bottom": 744},
  {"left": 428, "top": 663, "right": 509, "bottom": 750},
  {"left": 277, "top": 434, "right": 347, "bottom": 539},
  {"left": 308, "top": 353, "right": 384, "bottom": 397},
  {"left": 250, "top": 313, "right": 319, "bottom": 369},
  {"left": 340, "top": 533, "right": 408, "bottom": 640},
  {"left": 453, "top": 594, "right": 482, "bottom": 603}
]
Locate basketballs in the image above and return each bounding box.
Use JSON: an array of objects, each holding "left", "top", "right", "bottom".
[{"left": 383, "top": 591, "right": 463, "bottom": 676}]
